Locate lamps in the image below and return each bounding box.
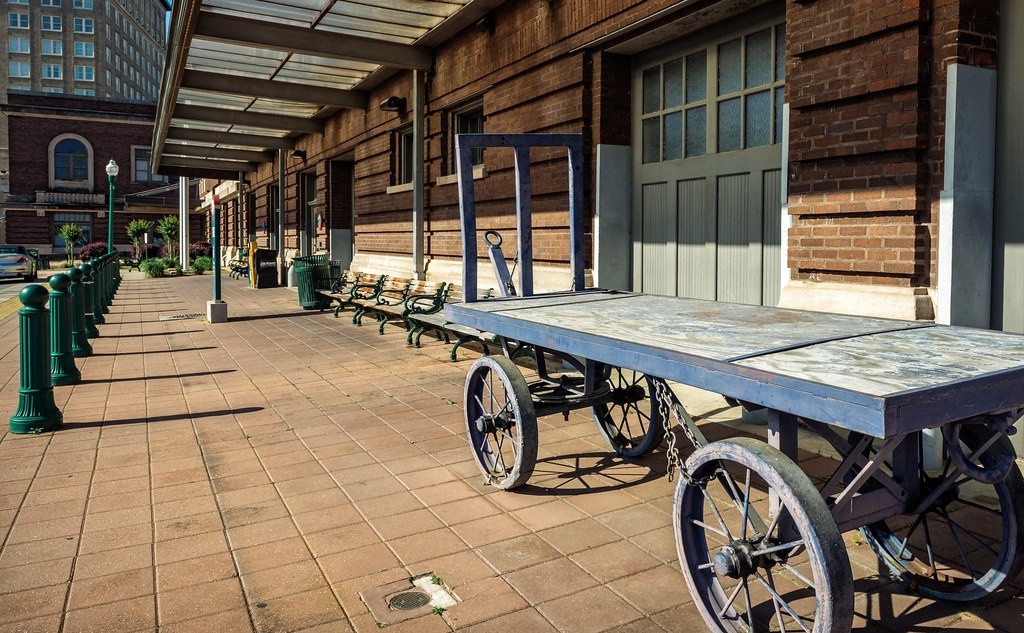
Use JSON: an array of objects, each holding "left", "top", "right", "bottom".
[
  {"left": 290, "top": 150, "right": 305, "bottom": 160},
  {"left": 379, "top": 96, "right": 406, "bottom": 112},
  {"left": 241, "top": 179, "right": 250, "bottom": 186}
]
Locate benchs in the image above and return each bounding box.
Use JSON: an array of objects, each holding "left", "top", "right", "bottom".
[
  {"left": 128, "top": 260, "right": 140, "bottom": 272},
  {"left": 320, "top": 269, "right": 536, "bottom": 381},
  {"left": 228, "top": 259, "right": 249, "bottom": 280}
]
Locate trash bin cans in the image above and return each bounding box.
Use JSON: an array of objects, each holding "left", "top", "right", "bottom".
[
  {"left": 329, "top": 260, "right": 342, "bottom": 290},
  {"left": 291, "top": 254, "right": 332, "bottom": 310}
]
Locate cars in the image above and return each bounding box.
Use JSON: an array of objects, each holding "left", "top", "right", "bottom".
[{"left": 0, "top": 245, "right": 38, "bottom": 282}]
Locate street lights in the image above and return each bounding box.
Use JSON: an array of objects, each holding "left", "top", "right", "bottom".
[{"left": 106, "top": 156, "right": 119, "bottom": 254}]
